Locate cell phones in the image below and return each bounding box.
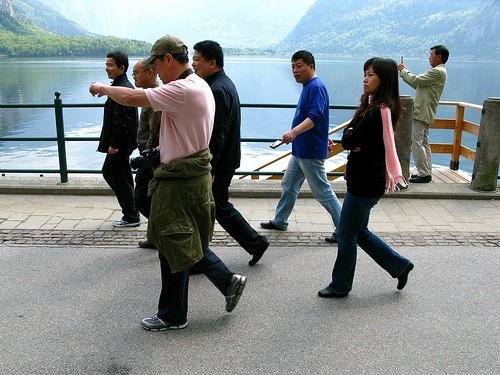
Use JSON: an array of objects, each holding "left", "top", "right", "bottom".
[
  {"left": 270, "top": 139, "right": 283, "bottom": 149},
  {"left": 401, "top": 56, "right": 404, "bottom": 63}
]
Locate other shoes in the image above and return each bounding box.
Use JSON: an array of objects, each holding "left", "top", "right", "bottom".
[
  {"left": 138, "top": 239, "right": 158, "bottom": 249},
  {"left": 318, "top": 286, "right": 348, "bottom": 297},
  {"left": 325, "top": 232, "right": 338, "bottom": 243},
  {"left": 260, "top": 221, "right": 285, "bottom": 231},
  {"left": 411, "top": 175, "right": 432, "bottom": 180},
  {"left": 408, "top": 177, "right": 431, "bottom": 183},
  {"left": 249, "top": 239, "right": 270, "bottom": 266},
  {"left": 396, "top": 263, "right": 414, "bottom": 290}
]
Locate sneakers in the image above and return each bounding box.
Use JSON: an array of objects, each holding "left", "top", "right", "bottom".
[
  {"left": 223, "top": 274, "right": 247, "bottom": 312},
  {"left": 113, "top": 218, "right": 140, "bottom": 227},
  {"left": 140, "top": 314, "right": 187, "bottom": 331}
]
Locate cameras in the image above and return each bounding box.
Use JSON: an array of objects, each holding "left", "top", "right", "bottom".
[{"left": 130, "top": 147, "right": 160, "bottom": 171}]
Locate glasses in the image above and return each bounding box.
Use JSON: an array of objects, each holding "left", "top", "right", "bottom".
[{"left": 132, "top": 67, "right": 150, "bottom": 78}]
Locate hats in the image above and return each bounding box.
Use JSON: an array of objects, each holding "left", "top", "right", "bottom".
[{"left": 141, "top": 35, "right": 188, "bottom": 65}]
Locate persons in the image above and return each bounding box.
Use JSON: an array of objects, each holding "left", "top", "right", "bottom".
[
  {"left": 188, "top": 40, "right": 270, "bottom": 275},
  {"left": 399, "top": 45, "right": 449, "bottom": 183},
  {"left": 317, "top": 57, "right": 414, "bottom": 298},
  {"left": 89, "top": 34, "right": 248, "bottom": 333},
  {"left": 132, "top": 57, "right": 162, "bottom": 250},
  {"left": 260, "top": 50, "right": 343, "bottom": 242},
  {"left": 96, "top": 50, "right": 141, "bottom": 228}
]
